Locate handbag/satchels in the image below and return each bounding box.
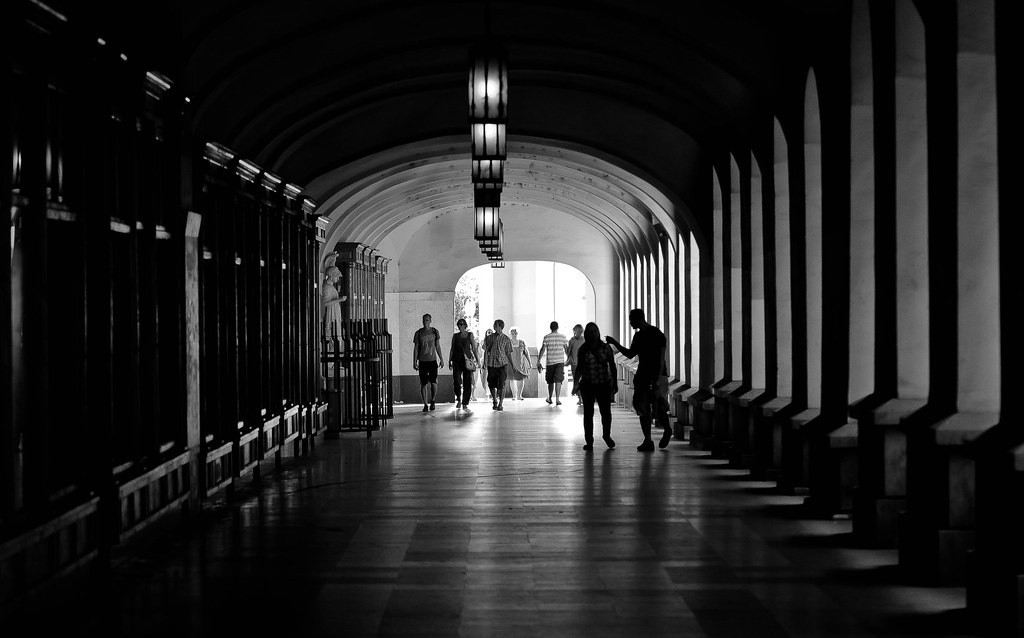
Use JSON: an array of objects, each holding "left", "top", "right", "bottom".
[{"left": 465, "top": 355, "right": 477, "bottom": 371}]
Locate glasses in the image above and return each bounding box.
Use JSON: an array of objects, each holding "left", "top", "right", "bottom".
[{"left": 458, "top": 322, "right": 465, "bottom": 326}]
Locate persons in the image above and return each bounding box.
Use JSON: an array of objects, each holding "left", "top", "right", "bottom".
[
  {"left": 571, "top": 322, "right": 619, "bottom": 450},
  {"left": 506, "top": 329, "right": 533, "bottom": 400},
  {"left": 413, "top": 313, "right": 444, "bottom": 412},
  {"left": 448, "top": 318, "right": 481, "bottom": 409},
  {"left": 482, "top": 319, "right": 517, "bottom": 411},
  {"left": 564, "top": 324, "right": 585, "bottom": 405},
  {"left": 536, "top": 321, "right": 568, "bottom": 405},
  {"left": 322, "top": 266, "right": 348, "bottom": 370},
  {"left": 605, "top": 308, "right": 673, "bottom": 452}
]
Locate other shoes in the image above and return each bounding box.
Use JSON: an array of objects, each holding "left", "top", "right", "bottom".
[
  {"left": 546, "top": 398, "right": 552, "bottom": 404},
  {"left": 463, "top": 405, "right": 467, "bottom": 409},
  {"left": 557, "top": 401, "right": 561, "bottom": 405},
  {"left": 493, "top": 400, "right": 503, "bottom": 410},
  {"left": 430, "top": 405, "right": 435, "bottom": 410},
  {"left": 601, "top": 436, "right": 616, "bottom": 449},
  {"left": 583, "top": 445, "right": 593, "bottom": 451},
  {"left": 423, "top": 405, "right": 428, "bottom": 411},
  {"left": 638, "top": 442, "right": 654, "bottom": 452},
  {"left": 456, "top": 402, "right": 460, "bottom": 408},
  {"left": 659, "top": 430, "right": 672, "bottom": 448}
]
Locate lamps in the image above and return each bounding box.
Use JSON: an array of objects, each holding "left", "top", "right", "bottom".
[{"left": 464, "top": 51, "right": 511, "bottom": 269}]
